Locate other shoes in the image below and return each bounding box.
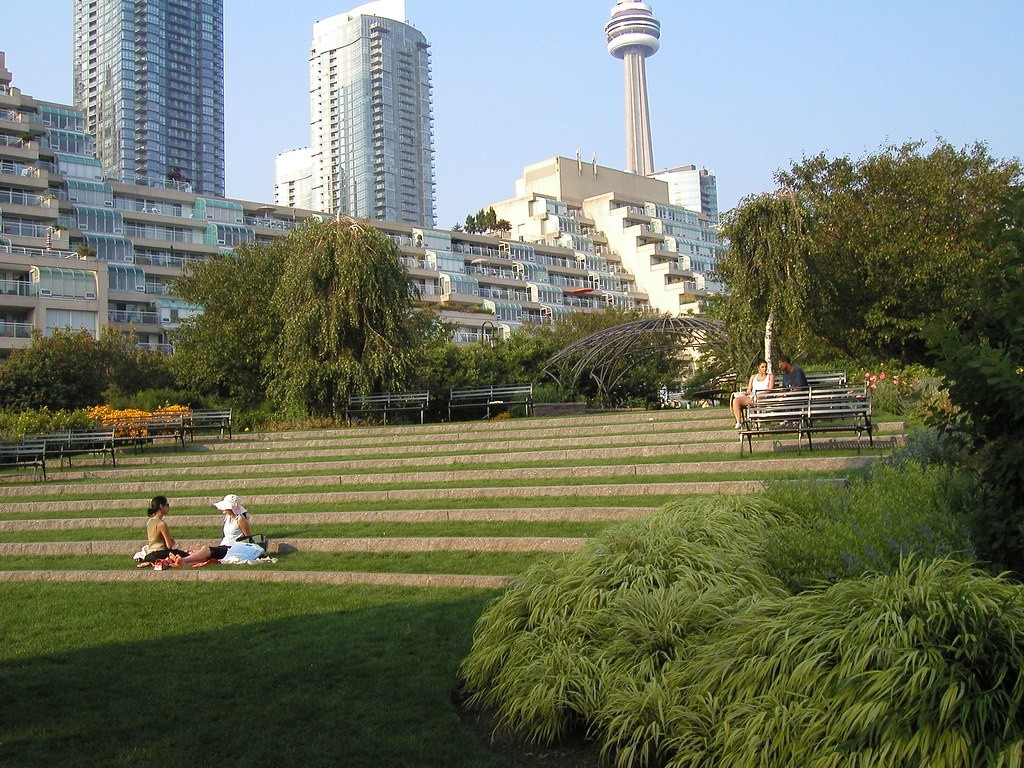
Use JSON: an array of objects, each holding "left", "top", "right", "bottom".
[
  {"left": 247, "top": 556, "right": 278, "bottom": 565},
  {"left": 792, "top": 421, "right": 799, "bottom": 428},
  {"left": 754, "top": 423, "right": 761, "bottom": 427},
  {"left": 137, "top": 561, "right": 151, "bottom": 567},
  {"left": 735, "top": 422, "right": 741, "bottom": 429},
  {"left": 154, "top": 559, "right": 167, "bottom": 570},
  {"left": 778, "top": 421, "right": 789, "bottom": 427}
]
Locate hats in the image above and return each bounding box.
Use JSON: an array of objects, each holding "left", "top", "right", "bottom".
[{"left": 213, "top": 495, "right": 247, "bottom": 515}]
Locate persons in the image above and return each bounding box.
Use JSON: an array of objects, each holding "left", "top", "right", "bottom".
[
  {"left": 146, "top": 496, "right": 175, "bottom": 551},
  {"left": 169, "top": 494, "right": 251, "bottom": 566},
  {"left": 733, "top": 355, "right": 809, "bottom": 430}
]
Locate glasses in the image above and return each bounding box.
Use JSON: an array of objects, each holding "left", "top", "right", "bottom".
[{"left": 164, "top": 504, "right": 169, "bottom": 507}]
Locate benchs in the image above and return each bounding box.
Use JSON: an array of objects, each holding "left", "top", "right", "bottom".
[
  {"left": 91, "top": 414, "right": 185, "bottom": 458},
  {"left": 730, "top": 369, "right": 872, "bottom": 458},
  {"left": 0, "top": 440, "right": 47, "bottom": 486},
  {"left": 16, "top": 428, "right": 116, "bottom": 472},
  {"left": 692, "top": 370, "right": 739, "bottom": 408},
  {"left": 145, "top": 408, "right": 233, "bottom": 446},
  {"left": 444, "top": 382, "right": 534, "bottom": 422},
  {"left": 344, "top": 390, "right": 430, "bottom": 427}
]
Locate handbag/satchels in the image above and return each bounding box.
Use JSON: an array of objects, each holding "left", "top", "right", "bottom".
[{"left": 220, "top": 534, "right": 279, "bottom": 564}]
